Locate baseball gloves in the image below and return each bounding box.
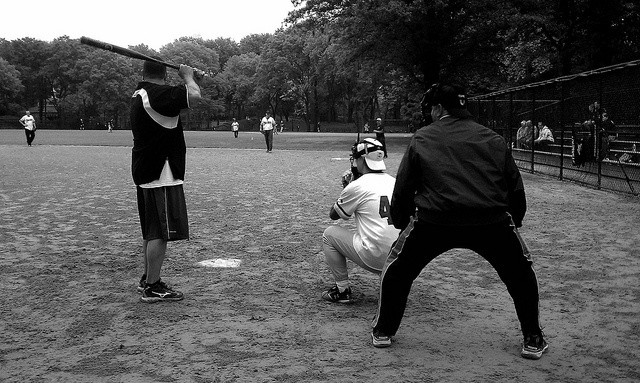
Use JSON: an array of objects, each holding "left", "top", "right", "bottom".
[{"left": 343, "top": 169, "right": 354, "bottom": 188}]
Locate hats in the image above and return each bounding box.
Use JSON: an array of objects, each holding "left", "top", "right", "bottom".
[
  {"left": 437, "top": 81, "right": 470, "bottom": 119},
  {"left": 356, "top": 138, "right": 387, "bottom": 171}
]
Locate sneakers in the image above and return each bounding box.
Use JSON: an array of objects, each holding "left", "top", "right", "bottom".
[
  {"left": 521, "top": 334, "right": 550, "bottom": 360},
  {"left": 321, "top": 285, "right": 353, "bottom": 303},
  {"left": 137, "top": 277, "right": 146, "bottom": 292},
  {"left": 369, "top": 327, "right": 392, "bottom": 348},
  {"left": 141, "top": 279, "right": 184, "bottom": 301}
]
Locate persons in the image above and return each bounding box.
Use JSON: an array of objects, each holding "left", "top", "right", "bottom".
[
  {"left": 279, "top": 120, "right": 285, "bottom": 133},
  {"left": 107, "top": 118, "right": 115, "bottom": 132},
  {"left": 369, "top": 85, "right": 549, "bottom": 360},
  {"left": 363, "top": 121, "right": 370, "bottom": 133},
  {"left": 231, "top": 118, "right": 240, "bottom": 139},
  {"left": 129, "top": 55, "right": 201, "bottom": 304},
  {"left": 321, "top": 136, "right": 402, "bottom": 303},
  {"left": 372, "top": 117, "right": 388, "bottom": 158},
  {"left": 316, "top": 121, "right": 320, "bottom": 132},
  {"left": 570, "top": 100, "right": 621, "bottom": 169},
  {"left": 259, "top": 110, "right": 276, "bottom": 153},
  {"left": 79, "top": 117, "right": 85, "bottom": 130},
  {"left": 516, "top": 119, "right": 555, "bottom": 153},
  {"left": 19, "top": 111, "right": 37, "bottom": 146}
]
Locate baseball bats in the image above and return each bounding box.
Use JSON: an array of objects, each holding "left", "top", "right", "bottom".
[{"left": 81, "top": 36, "right": 205, "bottom": 80}]
